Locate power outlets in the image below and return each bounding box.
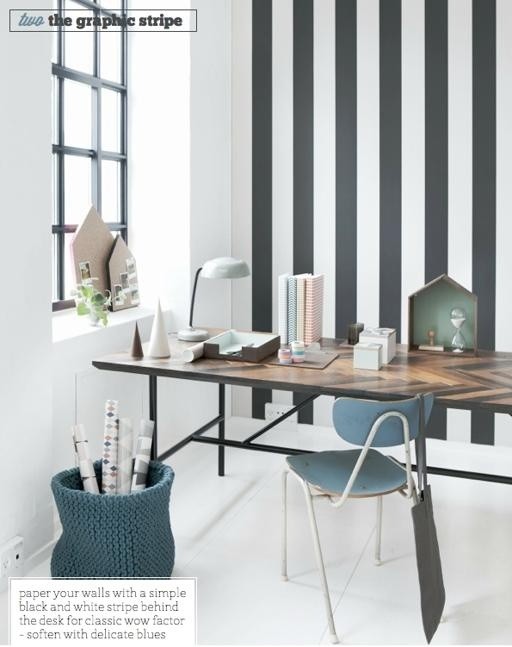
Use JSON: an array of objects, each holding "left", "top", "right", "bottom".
[{"left": 265, "top": 401, "right": 298, "bottom": 424}]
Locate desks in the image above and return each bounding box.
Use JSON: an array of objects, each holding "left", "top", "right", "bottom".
[{"left": 91, "top": 326, "right": 512, "bottom": 484}]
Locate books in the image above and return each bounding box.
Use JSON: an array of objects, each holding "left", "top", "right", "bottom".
[
  {"left": 277, "top": 273, "right": 288, "bottom": 346},
  {"left": 297, "top": 273, "right": 312, "bottom": 346},
  {"left": 288, "top": 272, "right": 303, "bottom": 346},
  {"left": 305, "top": 272, "right": 323, "bottom": 346}
]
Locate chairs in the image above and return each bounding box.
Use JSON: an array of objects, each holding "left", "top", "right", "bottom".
[{"left": 281, "top": 392, "right": 435, "bottom": 643}]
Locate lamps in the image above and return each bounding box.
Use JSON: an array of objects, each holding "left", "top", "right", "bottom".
[{"left": 175, "top": 255, "right": 249, "bottom": 341}]
